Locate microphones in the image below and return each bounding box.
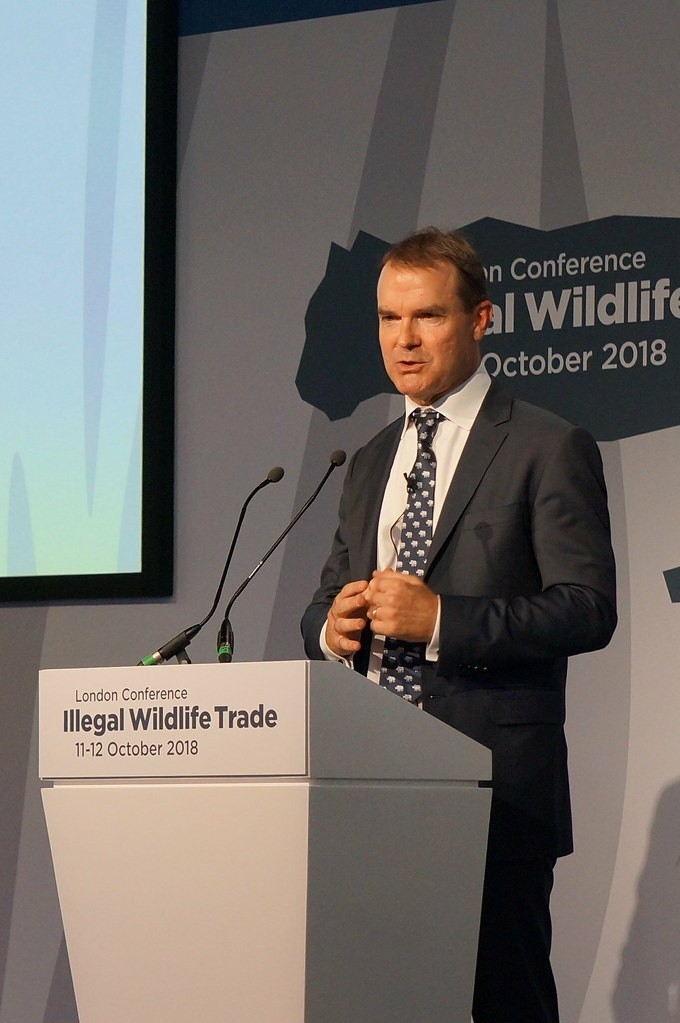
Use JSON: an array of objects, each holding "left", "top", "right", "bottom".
[
  {"left": 216, "top": 449, "right": 347, "bottom": 664},
  {"left": 404, "top": 471, "right": 420, "bottom": 495},
  {"left": 136, "top": 467, "right": 283, "bottom": 668}
]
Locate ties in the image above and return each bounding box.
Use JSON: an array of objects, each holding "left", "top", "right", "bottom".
[{"left": 378, "top": 410, "right": 446, "bottom": 705}]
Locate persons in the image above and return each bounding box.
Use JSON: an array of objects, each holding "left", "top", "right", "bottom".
[{"left": 300, "top": 226, "right": 618, "bottom": 1023}]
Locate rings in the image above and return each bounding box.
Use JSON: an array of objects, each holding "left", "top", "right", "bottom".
[{"left": 371, "top": 606, "right": 383, "bottom": 620}]
